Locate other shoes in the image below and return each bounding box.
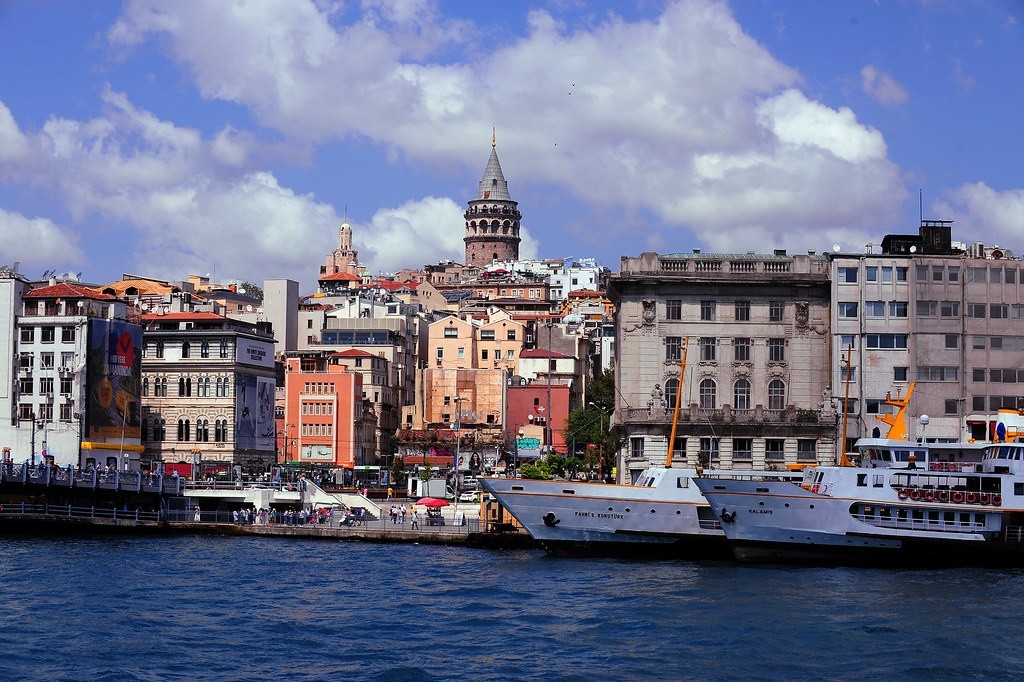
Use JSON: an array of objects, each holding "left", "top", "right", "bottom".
[
  {"left": 417, "top": 528, "right": 418, "bottom": 530},
  {"left": 364, "top": 524, "right": 365, "bottom": 526},
  {"left": 411, "top": 528, "right": 414, "bottom": 530}
]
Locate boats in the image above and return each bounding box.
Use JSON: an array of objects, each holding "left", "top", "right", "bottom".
[
  {"left": 690, "top": 406, "right": 1024, "bottom": 568},
  {"left": 474, "top": 334, "right": 826, "bottom": 560}
]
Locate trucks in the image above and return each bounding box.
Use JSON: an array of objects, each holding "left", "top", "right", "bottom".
[{"left": 407, "top": 477, "right": 459, "bottom": 502}]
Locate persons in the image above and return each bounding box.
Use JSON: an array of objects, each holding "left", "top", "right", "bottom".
[
  {"left": 95, "top": 461, "right": 104, "bottom": 479},
  {"left": 194, "top": 506, "right": 201, "bottom": 525},
  {"left": 385, "top": 485, "right": 394, "bottom": 505},
  {"left": 469, "top": 452, "right": 483, "bottom": 471},
  {"left": 389, "top": 504, "right": 406, "bottom": 525},
  {"left": 363, "top": 486, "right": 368, "bottom": 497},
  {"left": 233, "top": 504, "right": 364, "bottom": 528},
  {"left": 146, "top": 468, "right": 154, "bottom": 488},
  {"left": 411, "top": 507, "right": 419, "bottom": 530}
]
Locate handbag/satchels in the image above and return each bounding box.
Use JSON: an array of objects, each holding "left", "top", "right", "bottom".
[{"left": 411, "top": 520, "right": 413, "bottom": 524}]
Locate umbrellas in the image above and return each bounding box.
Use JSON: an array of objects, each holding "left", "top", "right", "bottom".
[{"left": 415, "top": 498, "right": 450, "bottom": 516}]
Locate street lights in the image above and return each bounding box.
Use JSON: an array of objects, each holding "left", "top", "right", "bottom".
[
  {"left": 27, "top": 353, "right": 48, "bottom": 463},
  {"left": 454, "top": 395, "right": 469, "bottom": 509},
  {"left": 588, "top": 402, "right": 604, "bottom": 481}
]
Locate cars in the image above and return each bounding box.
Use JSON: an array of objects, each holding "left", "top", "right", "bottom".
[{"left": 460, "top": 491, "right": 482, "bottom": 502}]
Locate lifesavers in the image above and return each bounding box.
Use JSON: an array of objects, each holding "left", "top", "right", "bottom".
[
  {"left": 947, "top": 462, "right": 958, "bottom": 472},
  {"left": 992, "top": 495, "right": 1002, "bottom": 505},
  {"left": 961, "top": 461, "right": 970, "bottom": 467},
  {"left": 953, "top": 493, "right": 964, "bottom": 503},
  {"left": 979, "top": 495, "right": 990, "bottom": 505},
  {"left": 910, "top": 490, "right": 921, "bottom": 500},
  {"left": 898, "top": 489, "right": 908, "bottom": 500},
  {"left": 924, "top": 490, "right": 935, "bottom": 500},
  {"left": 932, "top": 462, "right": 942, "bottom": 472},
  {"left": 938, "top": 492, "right": 949, "bottom": 502},
  {"left": 966, "top": 493, "right": 976, "bottom": 502}
]
